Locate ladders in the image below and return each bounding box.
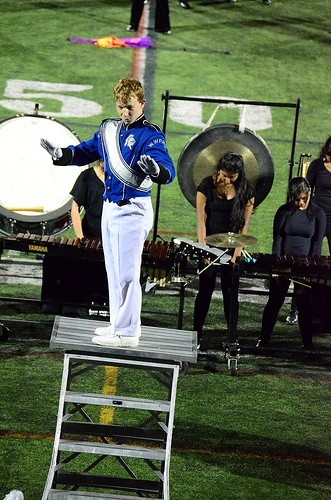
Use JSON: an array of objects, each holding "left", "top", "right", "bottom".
[{"left": 38, "top": 314, "right": 199, "bottom": 499}]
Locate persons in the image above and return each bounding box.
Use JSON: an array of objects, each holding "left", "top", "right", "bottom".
[
  {"left": 254, "top": 177, "right": 326, "bottom": 357},
  {"left": 70, "top": 159, "right": 106, "bottom": 239},
  {"left": 39, "top": 74, "right": 177, "bottom": 347},
  {"left": 193, "top": 152, "right": 255, "bottom": 352},
  {"left": 126, "top": 0, "right": 172, "bottom": 36},
  {"left": 306, "top": 136, "right": 331, "bottom": 258}
]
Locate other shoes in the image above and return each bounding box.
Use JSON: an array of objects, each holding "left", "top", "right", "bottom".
[
  {"left": 92, "top": 324, "right": 141, "bottom": 348},
  {"left": 126, "top": 25, "right": 138, "bottom": 31},
  {"left": 284, "top": 308, "right": 300, "bottom": 325},
  {"left": 162, "top": 27, "right": 171, "bottom": 35},
  {"left": 256, "top": 339, "right": 265, "bottom": 352},
  {"left": 306, "top": 348, "right": 320, "bottom": 365}
]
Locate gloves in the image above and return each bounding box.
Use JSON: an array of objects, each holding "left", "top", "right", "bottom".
[
  {"left": 39, "top": 137, "right": 62, "bottom": 158},
  {"left": 137, "top": 155, "right": 160, "bottom": 178}
]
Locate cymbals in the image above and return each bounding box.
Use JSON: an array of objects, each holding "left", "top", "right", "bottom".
[{"left": 206, "top": 232, "right": 259, "bottom": 248}]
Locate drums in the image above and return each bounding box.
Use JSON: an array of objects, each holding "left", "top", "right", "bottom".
[{"left": 0, "top": 114, "right": 90, "bottom": 238}]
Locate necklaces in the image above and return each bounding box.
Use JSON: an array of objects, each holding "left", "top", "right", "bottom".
[{"left": 222, "top": 185, "right": 233, "bottom": 194}]
